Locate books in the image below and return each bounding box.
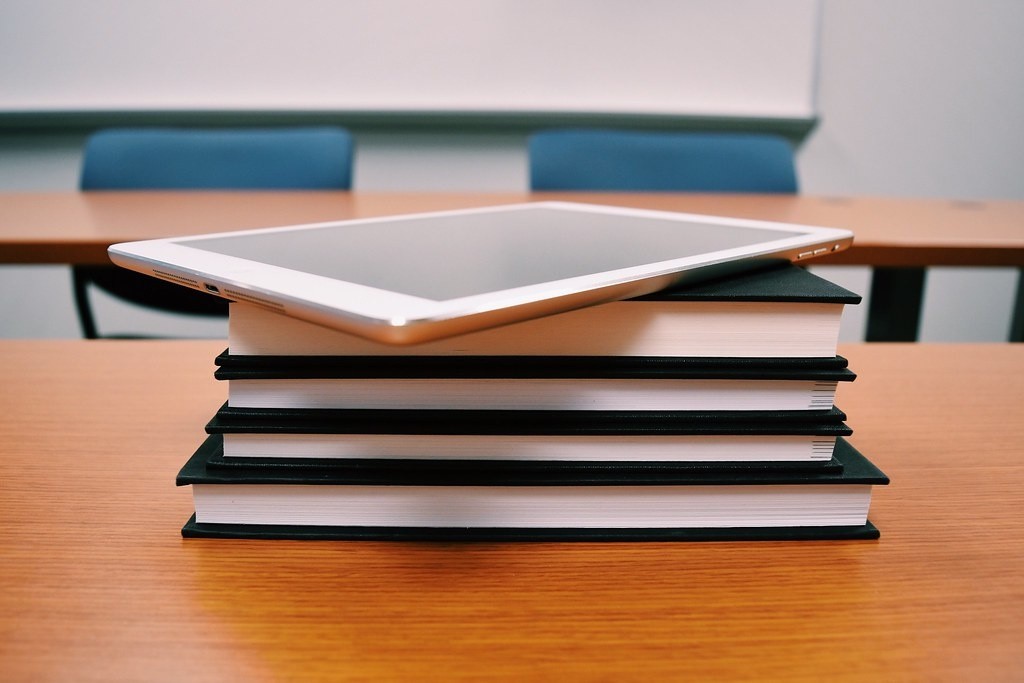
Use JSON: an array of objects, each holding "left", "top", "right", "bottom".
[{"left": 177, "top": 258, "right": 891, "bottom": 541}]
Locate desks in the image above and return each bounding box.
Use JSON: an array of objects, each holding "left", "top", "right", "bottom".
[
  {"left": 1, "top": 191, "right": 1024, "bottom": 345},
  {"left": 1, "top": 337, "right": 1024, "bottom": 683}
]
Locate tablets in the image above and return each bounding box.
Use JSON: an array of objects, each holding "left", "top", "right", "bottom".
[{"left": 105, "top": 202, "right": 854, "bottom": 347}]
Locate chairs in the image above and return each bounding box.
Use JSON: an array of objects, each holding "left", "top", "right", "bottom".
[
  {"left": 529, "top": 133, "right": 800, "bottom": 196},
  {"left": 72, "top": 124, "right": 356, "bottom": 334}
]
